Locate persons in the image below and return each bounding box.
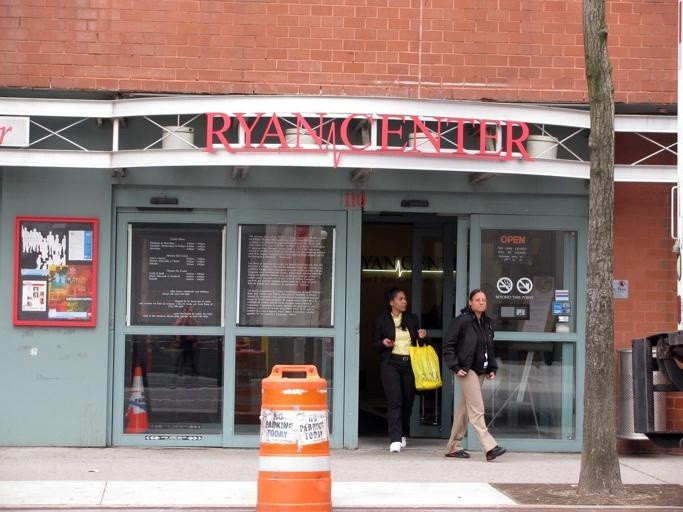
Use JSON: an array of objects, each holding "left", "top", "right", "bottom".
[
  {"left": 443, "top": 289, "right": 508, "bottom": 459},
  {"left": 374, "top": 287, "right": 427, "bottom": 452}
]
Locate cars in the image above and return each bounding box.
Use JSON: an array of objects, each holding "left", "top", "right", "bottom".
[{"left": 482, "top": 351, "right": 576, "bottom": 436}]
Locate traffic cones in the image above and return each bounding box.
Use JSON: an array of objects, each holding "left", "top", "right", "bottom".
[{"left": 126, "top": 367, "right": 149, "bottom": 433}]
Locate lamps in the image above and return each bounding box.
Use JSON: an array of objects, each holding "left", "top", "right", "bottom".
[
  {"left": 161, "top": 116, "right": 194, "bottom": 150},
  {"left": 407, "top": 121, "right": 441, "bottom": 150},
  {"left": 525, "top": 125, "right": 559, "bottom": 160},
  {"left": 285, "top": 119, "right": 318, "bottom": 145}
]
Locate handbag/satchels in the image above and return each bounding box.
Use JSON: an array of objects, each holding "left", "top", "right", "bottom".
[{"left": 407, "top": 338, "right": 442, "bottom": 391}]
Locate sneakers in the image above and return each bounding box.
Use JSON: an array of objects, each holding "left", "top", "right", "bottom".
[
  {"left": 390, "top": 437, "right": 407, "bottom": 452},
  {"left": 445, "top": 450, "right": 470, "bottom": 458},
  {"left": 487, "top": 445, "right": 507, "bottom": 460}
]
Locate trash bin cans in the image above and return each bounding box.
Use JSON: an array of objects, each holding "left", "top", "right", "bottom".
[{"left": 616, "top": 349, "right": 669, "bottom": 441}]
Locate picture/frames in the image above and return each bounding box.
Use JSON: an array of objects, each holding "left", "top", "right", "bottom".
[{"left": 12, "top": 215, "right": 99, "bottom": 329}]
